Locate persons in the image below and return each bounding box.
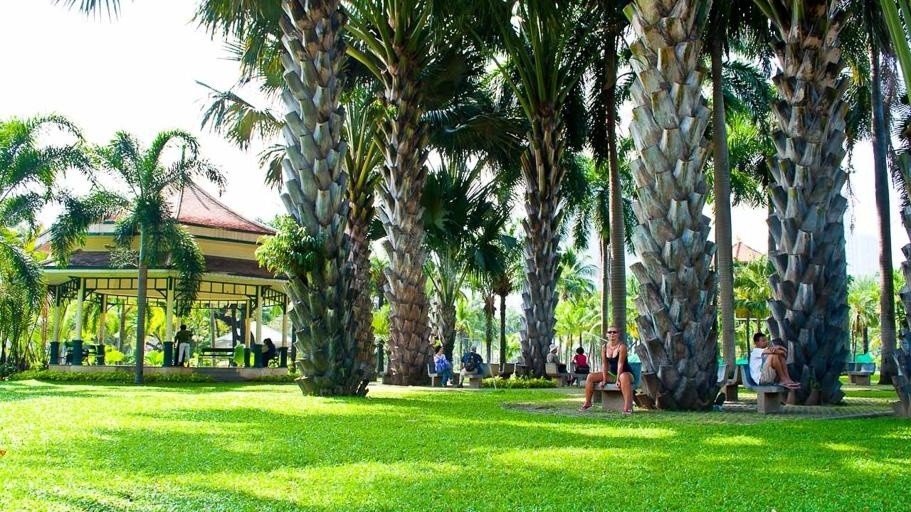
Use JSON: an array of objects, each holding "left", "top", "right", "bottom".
[
  {"left": 571, "top": 348, "right": 590, "bottom": 374},
  {"left": 456, "top": 344, "right": 484, "bottom": 388},
  {"left": 546, "top": 343, "right": 568, "bottom": 373},
  {"left": 174, "top": 324, "right": 193, "bottom": 368},
  {"left": 433, "top": 345, "right": 454, "bottom": 387},
  {"left": 261, "top": 338, "right": 277, "bottom": 367},
  {"left": 749, "top": 333, "right": 801, "bottom": 390},
  {"left": 579, "top": 326, "right": 636, "bottom": 415}
]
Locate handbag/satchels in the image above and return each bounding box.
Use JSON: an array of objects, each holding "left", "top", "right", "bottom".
[
  {"left": 575, "top": 366, "right": 589, "bottom": 374},
  {"left": 436, "top": 359, "right": 446, "bottom": 374}
]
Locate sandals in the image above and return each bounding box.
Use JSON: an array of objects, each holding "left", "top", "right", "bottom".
[
  {"left": 579, "top": 404, "right": 592, "bottom": 411},
  {"left": 779, "top": 381, "right": 801, "bottom": 389},
  {"left": 624, "top": 409, "right": 632, "bottom": 415}
]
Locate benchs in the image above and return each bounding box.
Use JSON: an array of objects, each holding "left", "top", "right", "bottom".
[
  {"left": 198, "top": 348, "right": 234, "bottom": 367},
  {"left": 427, "top": 363, "right": 460, "bottom": 387},
  {"left": 848, "top": 362, "right": 876, "bottom": 384}
]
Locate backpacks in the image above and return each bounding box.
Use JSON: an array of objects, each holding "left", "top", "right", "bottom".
[{"left": 465, "top": 355, "right": 475, "bottom": 372}]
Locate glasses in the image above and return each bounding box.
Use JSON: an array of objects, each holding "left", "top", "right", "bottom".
[{"left": 607, "top": 331, "right": 617, "bottom": 333}]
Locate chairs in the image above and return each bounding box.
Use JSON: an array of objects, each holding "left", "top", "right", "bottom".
[
  {"left": 498, "top": 363, "right": 513, "bottom": 386},
  {"left": 569, "top": 362, "right": 588, "bottom": 388},
  {"left": 740, "top": 363, "right": 795, "bottom": 414},
  {"left": 83, "top": 345, "right": 105, "bottom": 365},
  {"left": 268, "top": 348, "right": 281, "bottom": 368},
  {"left": 580, "top": 361, "right": 644, "bottom": 410},
  {"left": 545, "top": 362, "right": 567, "bottom": 386},
  {"left": 484, "top": 363, "right": 499, "bottom": 389},
  {"left": 463, "top": 362, "right": 483, "bottom": 388},
  {"left": 717, "top": 363, "right": 744, "bottom": 401}
]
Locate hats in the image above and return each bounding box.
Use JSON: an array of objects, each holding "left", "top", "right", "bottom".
[{"left": 549, "top": 344, "right": 558, "bottom": 352}]
